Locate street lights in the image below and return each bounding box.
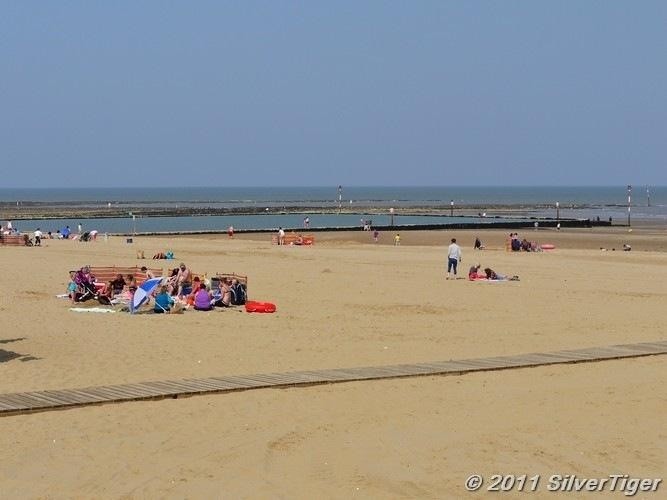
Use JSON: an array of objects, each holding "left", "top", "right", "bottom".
[
  {"left": 450, "top": 200, "right": 454, "bottom": 217},
  {"left": 555, "top": 201, "right": 560, "bottom": 219},
  {"left": 390, "top": 207, "right": 394, "bottom": 226}
]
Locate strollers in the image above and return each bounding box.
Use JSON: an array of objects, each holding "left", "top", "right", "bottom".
[
  {"left": 68, "top": 270, "right": 100, "bottom": 302},
  {"left": 79, "top": 230, "right": 91, "bottom": 242},
  {"left": 24, "top": 234, "right": 33, "bottom": 246}
]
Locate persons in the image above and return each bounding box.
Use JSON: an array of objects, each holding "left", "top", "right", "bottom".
[
  {"left": 0, "top": 219, "right": 248, "bottom": 313},
  {"left": 557, "top": 223, "right": 560, "bottom": 232},
  {"left": 276, "top": 226, "right": 285, "bottom": 244},
  {"left": 469, "top": 262, "right": 481, "bottom": 278},
  {"left": 395, "top": 233, "right": 401, "bottom": 246},
  {"left": 609, "top": 216, "right": 612, "bottom": 222},
  {"left": 484, "top": 268, "right": 519, "bottom": 280},
  {"left": 446, "top": 238, "right": 462, "bottom": 280},
  {"left": 506, "top": 233, "right": 543, "bottom": 252},
  {"left": 623, "top": 244, "right": 631, "bottom": 251},
  {"left": 304, "top": 216, "right": 309, "bottom": 228},
  {"left": 290, "top": 234, "right": 303, "bottom": 245},
  {"left": 534, "top": 221, "right": 538, "bottom": 232},
  {"left": 473, "top": 237, "right": 483, "bottom": 250},
  {"left": 361, "top": 217, "right": 372, "bottom": 231},
  {"left": 373, "top": 228, "right": 378, "bottom": 242}
]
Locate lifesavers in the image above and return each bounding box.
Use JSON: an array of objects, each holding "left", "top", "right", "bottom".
[
  {"left": 245, "top": 301, "right": 277, "bottom": 313},
  {"left": 542, "top": 244, "right": 555, "bottom": 249}
]
